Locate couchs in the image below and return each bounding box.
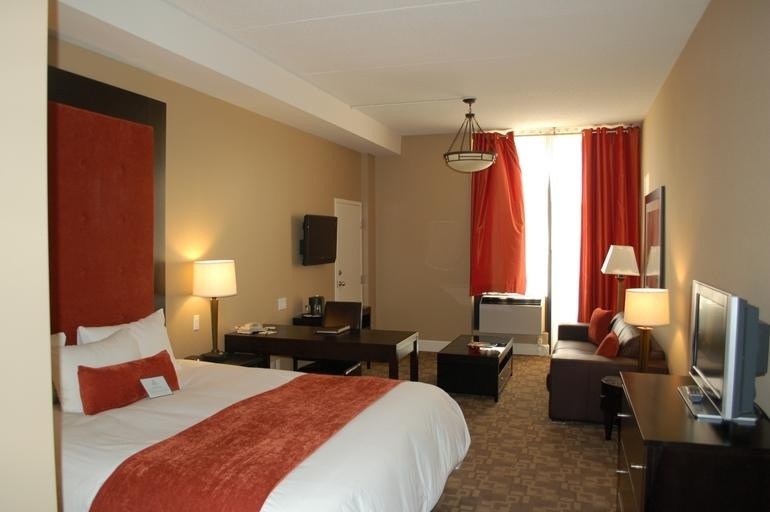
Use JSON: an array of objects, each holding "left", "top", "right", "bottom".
[{"left": 547, "top": 313, "right": 667, "bottom": 425}]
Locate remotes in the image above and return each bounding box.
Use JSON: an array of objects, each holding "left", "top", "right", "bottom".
[{"left": 688, "top": 385, "right": 704, "bottom": 402}]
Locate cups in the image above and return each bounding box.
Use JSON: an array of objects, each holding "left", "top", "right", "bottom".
[{"left": 304, "top": 305, "right": 310, "bottom": 313}]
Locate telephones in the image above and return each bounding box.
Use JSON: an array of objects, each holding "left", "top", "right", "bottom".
[{"left": 237, "top": 322, "right": 263, "bottom": 335}]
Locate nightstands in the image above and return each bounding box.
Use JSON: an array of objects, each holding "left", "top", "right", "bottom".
[{"left": 185, "top": 351, "right": 260, "bottom": 367}]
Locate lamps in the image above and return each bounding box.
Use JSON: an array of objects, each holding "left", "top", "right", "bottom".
[
  {"left": 624, "top": 288, "right": 670, "bottom": 372},
  {"left": 194, "top": 258, "right": 239, "bottom": 357},
  {"left": 444, "top": 97, "right": 498, "bottom": 172},
  {"left": 646, "top": 245, "right": 660, "bottom": 287},
  {"left": 603, "top": 244, "right": 637, "bottom": 308}
]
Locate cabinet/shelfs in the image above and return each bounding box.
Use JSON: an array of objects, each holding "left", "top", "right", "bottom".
[{"left": 614, "top": 373, "right": 770, "bottom": 512}]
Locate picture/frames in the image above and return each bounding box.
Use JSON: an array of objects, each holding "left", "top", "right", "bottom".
[{"left": 643, "top": 184, "right": 664, "bottom": 288}]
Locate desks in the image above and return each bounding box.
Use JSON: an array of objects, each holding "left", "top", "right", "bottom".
[
  {"left": 224, "top": 323, "right": 419, "bottom": 383},
  {"left": 292, "top": 306, "right": 372, "bottom": 372},
  {"left": 600, "top": 376, "right": 623, "bottom": 441}
]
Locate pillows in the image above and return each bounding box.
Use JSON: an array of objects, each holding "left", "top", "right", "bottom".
[
  {"left": 594, "top": 330, "right": 619, "bottom": 357},
  {"left": 77, "top": 351, "right": 179, "bottom": 415},
  {"left": 52, "top": 332, "right": 67, "bottom": 358},
  {"left": 75, "top": 308, "right": 175, "bottom": 357},
  {"left": 588, "top": 307, "right": 612, "bottom": 343},
  {"left": 52, "top": 330, "right": 140, "bottom": 414}
]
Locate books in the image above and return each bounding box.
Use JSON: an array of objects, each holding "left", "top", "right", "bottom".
[{"left": 316, "top": 325, "right": 350, "bottom": 334}]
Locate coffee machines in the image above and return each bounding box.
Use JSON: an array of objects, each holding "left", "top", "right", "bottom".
[{"left": 309, "top": 296, "right": 325, "bottom": 316}]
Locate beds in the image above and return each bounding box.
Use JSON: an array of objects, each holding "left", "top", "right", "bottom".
[{"left": 51, "top": 310, "right": 473, "bottom": 512}]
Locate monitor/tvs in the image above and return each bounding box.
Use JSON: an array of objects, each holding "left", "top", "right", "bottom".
[
  {"left": 300, "top": 214, "right": 338, "bottom": 266},
  {"left": 677, "top": 279, "right": 770, "bottom": 422}
]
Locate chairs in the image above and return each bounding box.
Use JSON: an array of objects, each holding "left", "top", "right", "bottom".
[{"left": 299, "top": 301, "right": 363, "bottom": 376}]
